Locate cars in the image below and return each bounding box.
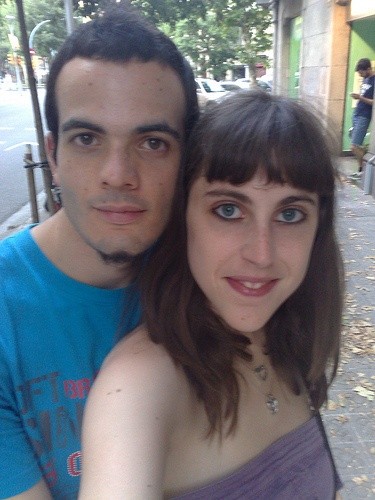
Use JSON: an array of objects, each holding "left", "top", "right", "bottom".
[{"left": 218, "top": 79, "right": 274, "bottom": 95}]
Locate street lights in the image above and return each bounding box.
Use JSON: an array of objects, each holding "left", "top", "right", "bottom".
[{"left": 5, "top": 15, "right": 23, "bottom": 90}]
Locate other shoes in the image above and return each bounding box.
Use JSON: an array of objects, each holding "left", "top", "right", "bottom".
[{"left": 347, "top": 172, "right": 363, "bottom": 179}]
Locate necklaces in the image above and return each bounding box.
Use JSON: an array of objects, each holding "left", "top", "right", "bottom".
[{"left": 254, "top": 366, "right": 280, "bottom": 415}]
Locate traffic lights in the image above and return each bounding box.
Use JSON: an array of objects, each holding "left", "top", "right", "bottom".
[
  {"left": 7, "top": 54, "right": 15, "bottom": 65},
  {"left": 16, "top": 54, "right": 25, "bottom": 67}
]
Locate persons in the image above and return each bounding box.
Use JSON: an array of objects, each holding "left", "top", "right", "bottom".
[
  {"left": 77, "top": 91, "right": 344, "bottom": 500},
  {"left": 349, "top": 58, "right": 375, "bottom": 172},
  {"left": 0, "top": 11, "right": 200, "bottom": 500}
]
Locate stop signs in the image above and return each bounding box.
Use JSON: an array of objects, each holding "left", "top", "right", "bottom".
[{"left": 28, "top": 48, "right": 36, "bottom": 57}]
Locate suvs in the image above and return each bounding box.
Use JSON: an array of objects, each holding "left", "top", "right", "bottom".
[{"left": 194, "top": 77, "right": 233, "bottom": 115}]
